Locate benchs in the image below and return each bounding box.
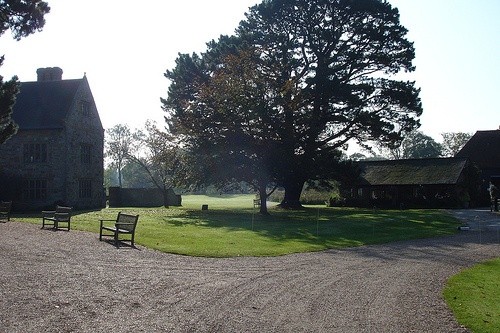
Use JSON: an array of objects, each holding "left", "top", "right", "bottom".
[
  {"left": 0, "top": 201, "right": 15, "bottom": 222},
  {"left": 99, "top": 211, "right": 139, "bottom": 247},
  {"left": 42, "top": 206, "right": 73, "bottom": 232}
]
